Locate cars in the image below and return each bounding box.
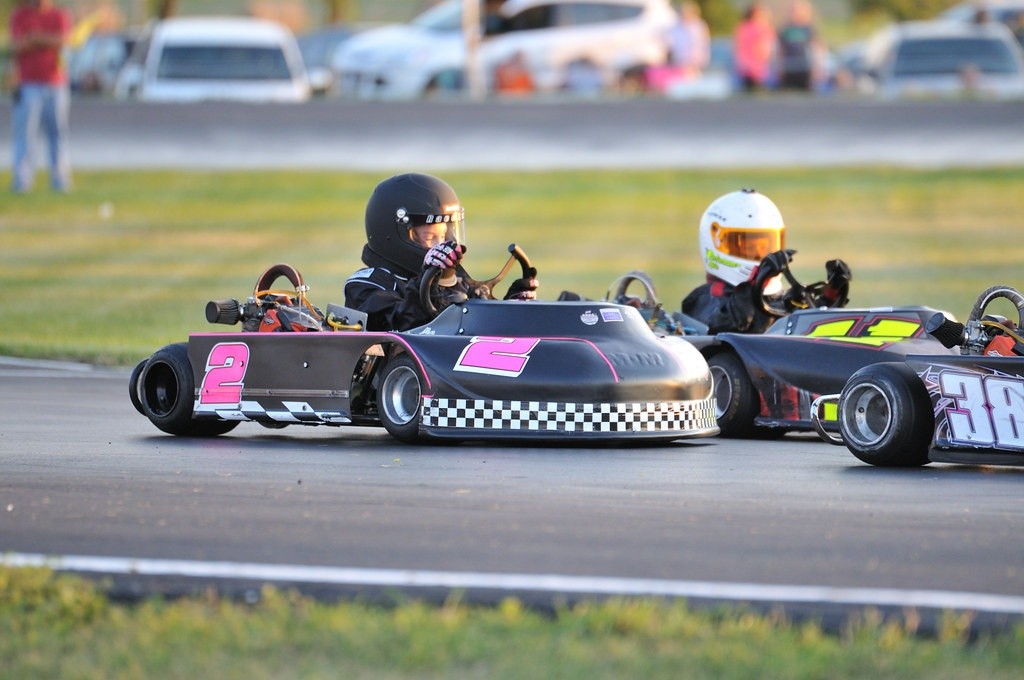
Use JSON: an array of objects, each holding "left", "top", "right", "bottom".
[{"left": 105, "top": 2, "right": 1024, "bottom": 100}]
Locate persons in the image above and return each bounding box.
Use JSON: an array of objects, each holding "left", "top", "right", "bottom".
[
  {"left": 9, "top": 0, "right": 80, "bottom": 196},
  {"left": 681, "top": 189, "right": 852, "bottom": 336},
  {"left": 492, "top": 44, "right": 658, "bottom": 101},
  {"left": 665, "top": 0, "right": 711, "bottom": 74},
  {"left": 730, "top": 0, "right": 828, "bottom": 93},
  {"left": 345, "top": 173, "right": 537, "bottom": 336}
]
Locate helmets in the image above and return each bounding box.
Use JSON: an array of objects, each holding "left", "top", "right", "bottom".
[
  {"left": 364, "top": 173, "right": 464, "bottom": 275},
  {"left": 698, "top": 189, "right": 786, "bottom": 287}
]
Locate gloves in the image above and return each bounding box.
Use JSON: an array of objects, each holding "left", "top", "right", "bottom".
[
  {"left": 749, "top": 249, "right": 796, "bottom": 291},
  {"left": 421, "top": 239, "right": 467, "bottom": 274},
  {"left": 824, "top": 261, "right": 852, "bottom": 293},
  {"left": 502, "top": 265, "right": 540, "bottom": 300}
]
